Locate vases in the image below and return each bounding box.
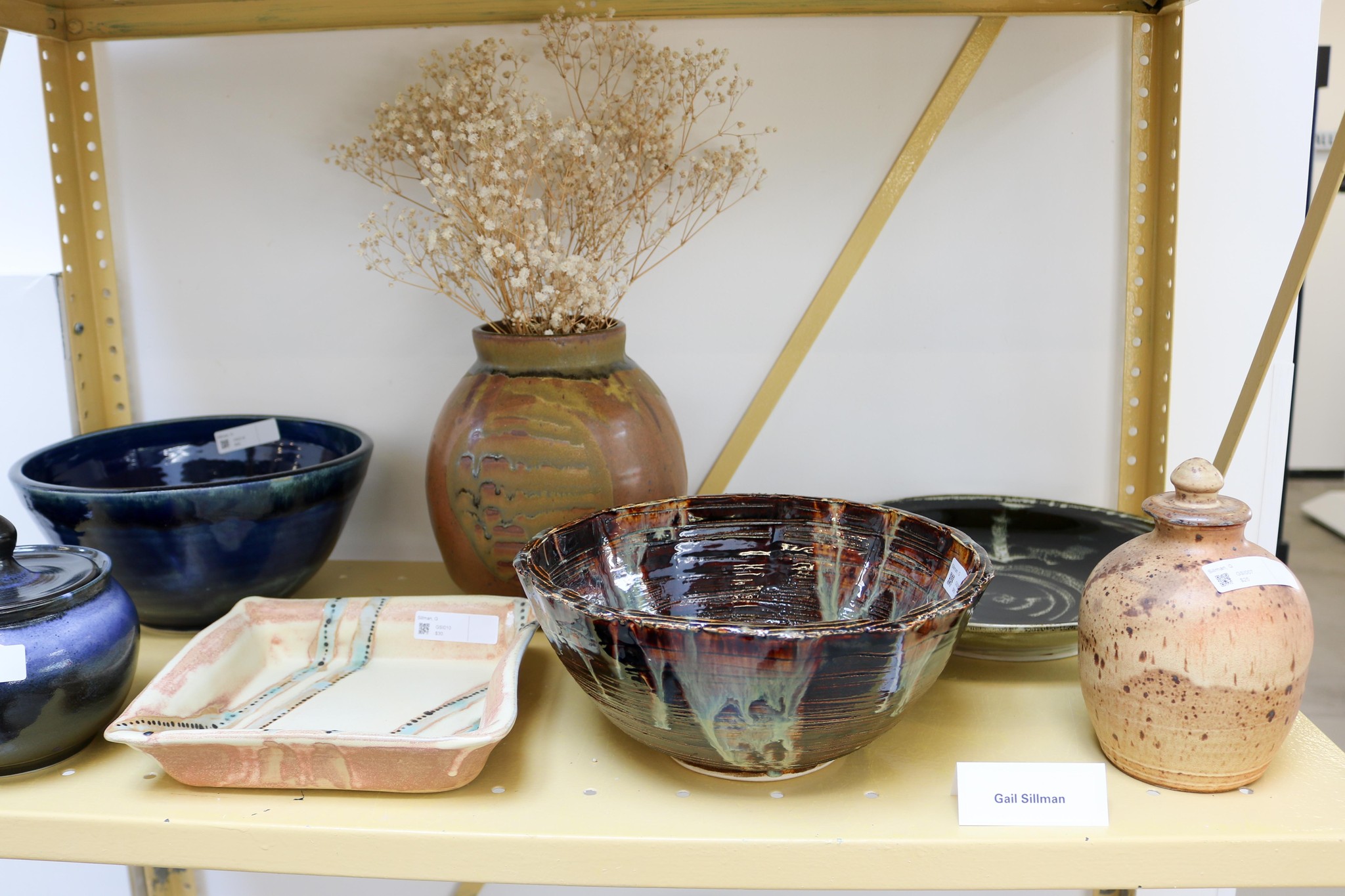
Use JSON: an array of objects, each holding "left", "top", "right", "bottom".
[{"left": 429, "top": 318, "right": 686, "bottom": 597}]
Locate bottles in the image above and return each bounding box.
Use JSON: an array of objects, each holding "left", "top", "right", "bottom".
[{"left": 1080, "top": 457, "right": 1312, "bottom": 791}]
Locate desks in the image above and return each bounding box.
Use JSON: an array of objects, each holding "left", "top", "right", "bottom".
[{"left": 0, "top": 555, "right": 1343, "bottom": 896}]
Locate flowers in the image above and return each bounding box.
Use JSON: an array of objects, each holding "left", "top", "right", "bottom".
[{"left": 323, "top": 3, "right": 777, "bottom": 339}]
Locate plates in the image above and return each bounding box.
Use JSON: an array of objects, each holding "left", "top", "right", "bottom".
[
  {"left": 99, "top": 585, "right": 549, "bottom": 791},
  {"left": 878, "top": 494, "right": 1166, "bottom": 663}
]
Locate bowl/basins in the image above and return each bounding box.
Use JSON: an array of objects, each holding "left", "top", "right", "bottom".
[
  {"left": 2, "top": 516, "right": 140, "bottom": 777},
  {"left": 6, "top": 414, "right": 375, "bottom": 631},
  {"left": 512, "top": 492, "right": 993, "bottom": 780}
]
